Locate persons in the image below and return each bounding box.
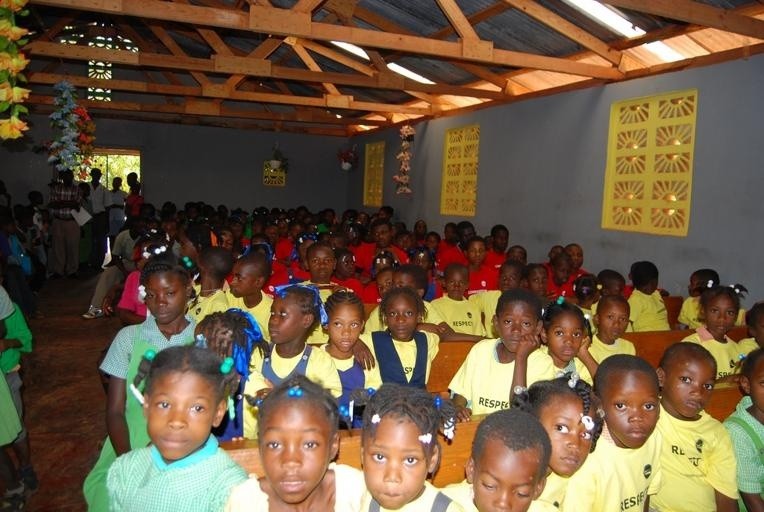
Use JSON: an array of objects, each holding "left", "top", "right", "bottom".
[
  {"left": 485, "top": 226, "right": 510, "bottom": 270},
  {"left": 106, "top": 345, "right": 248, "bottom": 512},
  {"left": 568, "top": 353, "right": 662, "bottom": 512},
  {"left": 201, "top": 202, "right": 222, "bottom": 227},
  {"left": 679, "top": 280, "right": 749, "bottom": 383},
  {"left": 540, "top": 301, "right": 600, "bottom": 388},
  {"left": 414, "top": 246, "right": 434, "bottom": 270},
  {"left": 657, "top": 341, "right": 739, "bottom": 512},
  {"left": 543, "top": 253, "right": 578, "bottom": 300},
  {"left": 115, "top": 228, "right": 175, "bottom": 324},
  {"left": 219, "top": 215, "right": 247, "bottom": 246},
  {"left": 138, "top": 200, "right": 158, "bottom": 221},
  {"left": 218, "top": 229, "right": 242, "bottom": 257},
  {"left": 354, "top": 218, "right": 410, "bottom": 266},
  {"left": 440, "top": 220, "right": 476, "bottom": 267},
  {"left": 411, "top": 217, "right": 428, "bottom": 248},
  {"left": 570, "top": 274, "right": 599, "bottom": 317},
  {"left": 544, "top": 244, "right": 564, "bottom": 259},
  {"left": 298, "top": 240, "right": 353, "bottom": 343},
  {"left": 723, "top": 348, "right": 764, "bottom": 512},
  {"left": 245, "top": 283, "right": 341, "bottom": 440},
  {"left": 81, "top": 218, "right": 146, "bottom": 320},
  {"left": 226, "top": 251, "right": 273, "bottom": 342},
  {"left": 253, "top": 201, "right": 316, "bottom": 222},
  {"left": 50, "top": 169, "right": 83, "bottom": 274},
  {"left": 426, "top": 262, "right": 486, "bottom": 336},
  {"left": 126, "top": 172, "right": 138, "bottom": 184},
  {"left": 591, "top": 294, "right": 637, "bottom": 364},
  {"left": 446, "top": 292, "right": 555, "bottom": 423},
  {"left": 328, "top": 223, "right": 342, "bottom": 231},
  {"left": 178, "top": 222, "right": 213, "bottom": 255},
  {"left": 459, "top": 236, "right": 499, "bottom": 289},
  {"left": 359, "top": 211, "right": 370, "bottom": 228},
  {"left": 105, "top": 178, "right": 124, "bottom": 268},
  {"left": 0, "top": 286, "right": 41, "bottom": 512},
  {"left": 422, "top": 230, "right": 441, "bottom": 256},
  {"left": 675, "top": 269, "right": 720, "bottom": 329},
  {"left": 342, "top": 222, "right": 360, "bottom": 246},
  {"left": 245, "top": 243, "right": 273, "bottom": 259},
  {"left": 565, "top": 243, "right": 589, "bottom": 275},
  {"left": 159, "top": 201, "right": 177, "bottom": 230},
  {"left": 1, "top": 303, "right": 37, "bottom": 495},
  {"left": 0, "top": 181, "right": 51, "bottom": 322},
  {"left": 218, "top": 205, "right": 229, "bottom": 216},
  {"left": 184, "top": 246, "right": 232, "bottom": 321},
  {"left": 595, "top": 268, "right": 626, "bottom": 296},
  {"left": 273, "top": 232, "right": 317, "bottom": 282},
  {"left": 262, "top": 223, "right": 280, "bottom": 244},
  {"left": 87, "top": 168, "right": 111, "bottom": 274},
  {"left": 237, "top": 212, "right": 253, "bottom": 236},
  {"left": 506, "top": 244, "right": 527, "bottom": 262},
  {"left": 350, "top": 287, "right": 440, "bottom": 400},
  {"left": 377, "top": 205, "right": 394, "bottom": 222},
  {"left": 321, "top": 288, "right": 383, "bottom": 429},
  {"left": 328, "top": 233, "right": 344, "bottom": 249},
  {"left": 333, "top": 247, "right": 359, "bottom": 288},
  {"left": 146, "top": 220, "right": 158, "bottom": 230},
  {"left": 226, "top": 374, "right": 365, "bottom": 512},
  {"left": 342, "top": 207, "right": 358, "bottom": 222},
  {"left": 194, "top": 309, "right": 269, "bottom": 443},
  {"left": 444, "top": 409, "right": 560, "bottom": 512},
  {"left": 352, "top": 385, "right": 468, "bottom": 512},
  {"left": 468, "top": 258, "right": 525, "bottom": 338},
  {"left": 85, "top": 256, "right": 198, "bottom": 512},
  {"left": 179, "top": 200, "right": 201, "bottom": 227},
  {"left": 625, "top": 261, "right": 671, "bottom": 332},
  {"left": 78, "top": 182, "right": 92, "bottom": 265},
  {"left": 247, "top": 233, "right": 270, "bottom": 249},
  {"left": 285, "top": 221, "right": 305, "bottom": 242},
  {"left": 524, "top": 261, "right": 548, "bottom": 295},
  {"left": 358, "top": 265, "right": 455, "bottom": 334},
  {"left": 125, "top": 182, "right": 144, "bottom": 221},
  {"left": 316, "top": 209, "right": 336, "bottom": 234},
  {"left": 392, "top": 227, "right": 411, "bottom": 251},
  {"left": 510, "top": 371, "right": 605, "bottom": 503},
  {"left": 738, "top": 302, "right": 764, "bottom": 360},
  {"left": 364, "top": 252, "right": 397, "bottom": 314},
  {"left": 318, "top": 231, "right": 331, "bottom": 248}
]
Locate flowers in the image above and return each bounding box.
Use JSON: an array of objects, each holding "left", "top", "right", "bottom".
[
  {"left": 392, "top": 124, "right": 416, "bottom": 199},
  {"left": 336, "top": 143, "right": 358, "bottom": 173},
  {"left": 32, "top": 79, "right": 96, "bottom": 187},
  {"left": 0, "top": 0, "right": 29, "bottom": 146}
]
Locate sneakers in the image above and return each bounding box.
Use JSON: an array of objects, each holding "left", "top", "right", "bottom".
[
  {"left": 0, "top": 483, "right": 27, "bottom": 512},
  {"left": 19, "top": 468, "right": 39, "bottom": 493},
  {"left": 82, "top": 306, "right": 104, "bottom": 320}
]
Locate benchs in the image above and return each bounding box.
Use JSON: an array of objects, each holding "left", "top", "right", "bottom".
[
  {"left": 427, "top": 326, "right": 759, "bottom": 405},
  {"left": 209, "top": 383, "right": 764, "bottom": 493}
]
